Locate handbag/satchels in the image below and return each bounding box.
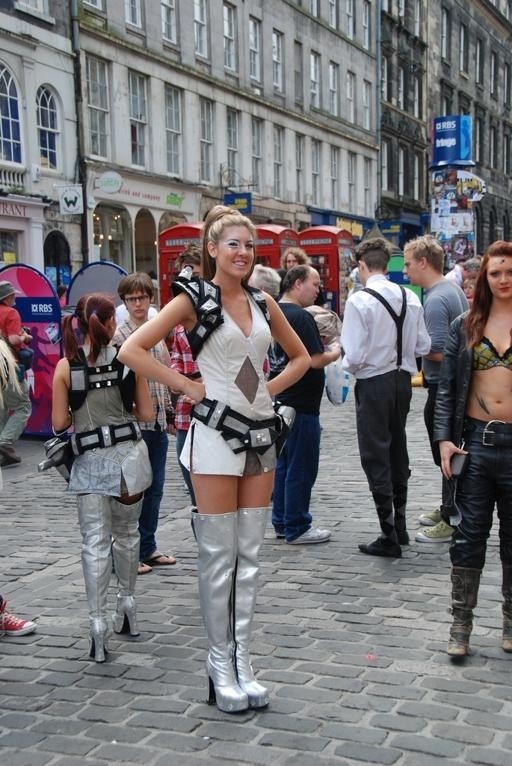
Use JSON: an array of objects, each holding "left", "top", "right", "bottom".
[{"left": 16, "top": 347, "right": 35, "bottom": 372}]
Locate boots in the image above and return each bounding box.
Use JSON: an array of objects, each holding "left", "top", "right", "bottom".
[
  {"left": 445, "top": 565, "right": 483, "bottom": 658},
  {"left": 110, "top": 491, "right": 145, "bottom": 637},
  {"left": 233, "top": 506, "right": 270, "bottom": 708},
  {"left": 501, "top": 562, "right": 512, "bottom": 654},
  {"left": 190, "top": 507, "right": 250, "bottom": 713},
  {"left": 76, "top": 493, "right": 112, "bottom": 662}
]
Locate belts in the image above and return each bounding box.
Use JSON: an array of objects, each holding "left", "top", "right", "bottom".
[{"left": 460, "top": 430, "right": 512, "bottom": 448}]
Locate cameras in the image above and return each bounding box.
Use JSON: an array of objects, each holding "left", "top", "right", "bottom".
[{"left": 23, "top": 326, "right": 31, "bottom": 344}]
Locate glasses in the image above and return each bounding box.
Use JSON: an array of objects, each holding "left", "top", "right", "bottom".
[{"left": 125, "top": 295, "right": 151, "bottom": 305}]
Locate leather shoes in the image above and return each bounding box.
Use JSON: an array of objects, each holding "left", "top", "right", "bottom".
[{"left": 357, "top": 530, "right": 410, "bottom": 559}]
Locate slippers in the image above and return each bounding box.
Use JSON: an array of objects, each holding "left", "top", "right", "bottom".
[{"left": 138, "top": 548, "right": 178, "bottom": 575}]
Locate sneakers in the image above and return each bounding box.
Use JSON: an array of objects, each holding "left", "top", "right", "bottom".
[
  {"left": 414, "top": 507, "right": 456, "bottom": 543},
  {"left": 0, "top": 600, "right": 39, "bottom": 639},
  {"left": 0, "top": 442, "right": 22, "bottom": 468},
  {"left": 276, "top": 526, "right": 333, "bottom": 545}
]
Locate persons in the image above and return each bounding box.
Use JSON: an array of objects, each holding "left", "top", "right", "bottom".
[
  {"left": 112, "top": 272, "right": 177, "bottom": 573},
  {"left": 166, "top": 243, "right": 200, "bottom": 542},
  {"left": 51, "top": 293, "right": 154, "bottom": 664},
  {"left": 0, "top": 279, "right": 34, "bottom": 468},
  {"left": 0, "top": 334, "right": 38, "bottom": 640},
  {"left": 434, "top": 240, "right": 512, "bottom": 656},
  {"left": 57, "top": 284, "right": 67, "bottom": 308},
  {"left": 117, "top": 204, "right": 311, "bottom": 713}
]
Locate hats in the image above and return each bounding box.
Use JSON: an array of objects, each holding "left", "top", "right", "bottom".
[{"left": 0, "top": 280, "right": 22, "bottom": 303}]
